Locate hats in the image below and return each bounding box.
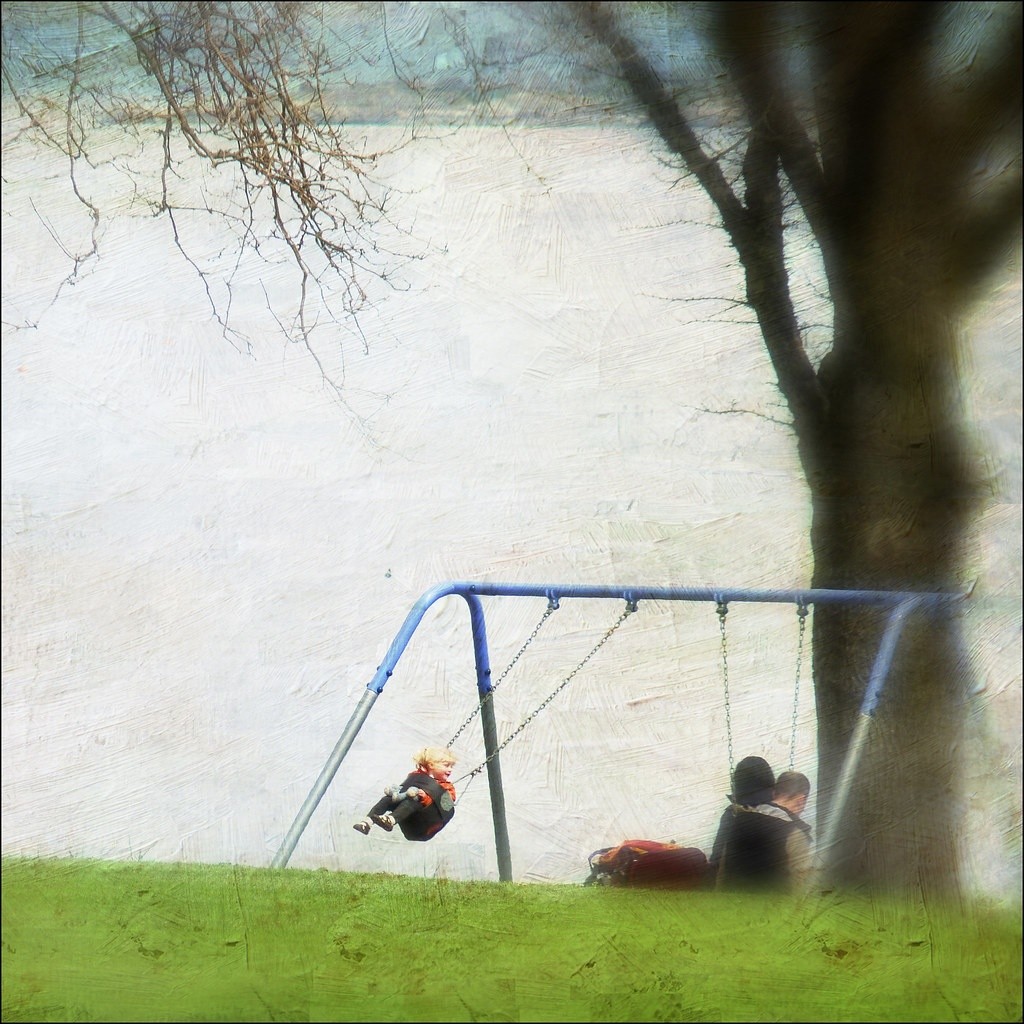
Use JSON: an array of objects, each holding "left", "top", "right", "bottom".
[{"left": 731, "top": 756, "right": 776, "bottom": 804}]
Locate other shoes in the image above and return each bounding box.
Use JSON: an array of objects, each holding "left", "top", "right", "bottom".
[
  {"left": 353, "top": 821, "right": 370, "bottom": 835},
  {"left": 370, "top": 813, "right": 393, "bottom": 831}
]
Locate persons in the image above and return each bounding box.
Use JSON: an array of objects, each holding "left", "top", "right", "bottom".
[
  {"left": 710, "top": 758, "right": 814, "bottom": 895},
  {"left": 352, "top": 746, "right": 456, "bottom": 841}
]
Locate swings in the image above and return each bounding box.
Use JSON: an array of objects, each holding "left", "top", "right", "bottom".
[
  {"left": 710, "top": 598, "right": 816, "bottom": 895},
  {"left": 393, "top": 596, "right": 641, "bottom": 843}
]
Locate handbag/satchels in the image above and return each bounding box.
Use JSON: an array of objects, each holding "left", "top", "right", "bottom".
[
  {"left": 588, "top": 839, "right": 683, "bottom": 873},
  {"left": 627, "top": 847, "right": 709, "bottom": 890}
]
